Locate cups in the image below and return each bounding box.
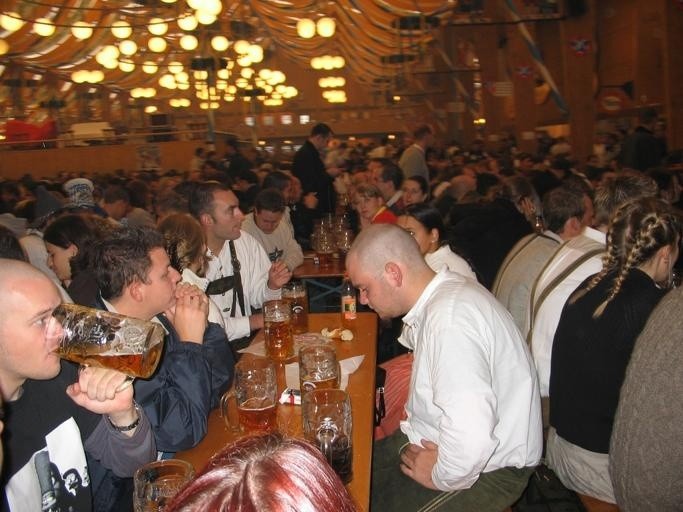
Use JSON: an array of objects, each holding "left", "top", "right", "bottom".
[
  {"left": 297, "top": 344, "right": 344, "bottom": 408},
  {"left": 300, "top": 388, "right": 354, "bottom": 484},
  {"left": 262, "top": 300, "right": 296, "bottom": 359},
  {"left": 315, "top": 216, "right": 354, "bottom": 268},
  {"left": 281, "top": 282, "right": 309, "bottom": 335},
  {"left": 219, "top": 358, "right": 278, "bottom": 444},
  {"left": 132, "top": 457, "right": 193, "bottom": 512},
  {"left": 43, "top": 303, "right": 166, "bottom": 381}
]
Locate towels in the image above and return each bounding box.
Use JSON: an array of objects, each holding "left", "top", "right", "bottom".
[
  {"left": 285, "top": 355, "right": 365, "bottom": 392},
  {"left": 237, "top": 333, "right": 332, "bottom": 358}
]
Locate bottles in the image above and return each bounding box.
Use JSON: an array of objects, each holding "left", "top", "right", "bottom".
[{"left": 339, "top": 273, "right": 358, "bottom": 329}]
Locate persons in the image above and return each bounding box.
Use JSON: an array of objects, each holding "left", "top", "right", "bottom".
[{"left": 1, "top": 104, "right": 682, "bottom": 511}]
